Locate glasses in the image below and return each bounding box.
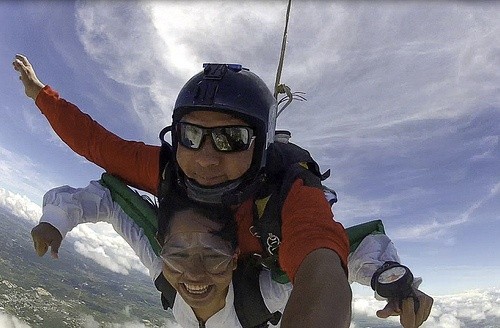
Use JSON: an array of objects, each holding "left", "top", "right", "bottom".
[
  {"left": 176, "top": 121, "right": 258, "bottom": 153},
  {"left": 157, "top": 230, "right": 237, "bottom": 275}
]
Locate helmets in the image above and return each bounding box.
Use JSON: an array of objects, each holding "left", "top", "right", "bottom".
[{"left": 172, "top": 62, "right": 277, "bottom": 205}]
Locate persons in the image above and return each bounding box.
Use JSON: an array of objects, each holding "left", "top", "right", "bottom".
[
  {"left": 30, "top": 176, "right": 434, "bottom": 328},
  {"left": 11, "top": 52, "right": 355, "bottom": 328}
]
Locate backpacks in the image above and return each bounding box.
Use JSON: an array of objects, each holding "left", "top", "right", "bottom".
[{"left": 157, "top": 138, "right": 336, "bottom": 278}]
